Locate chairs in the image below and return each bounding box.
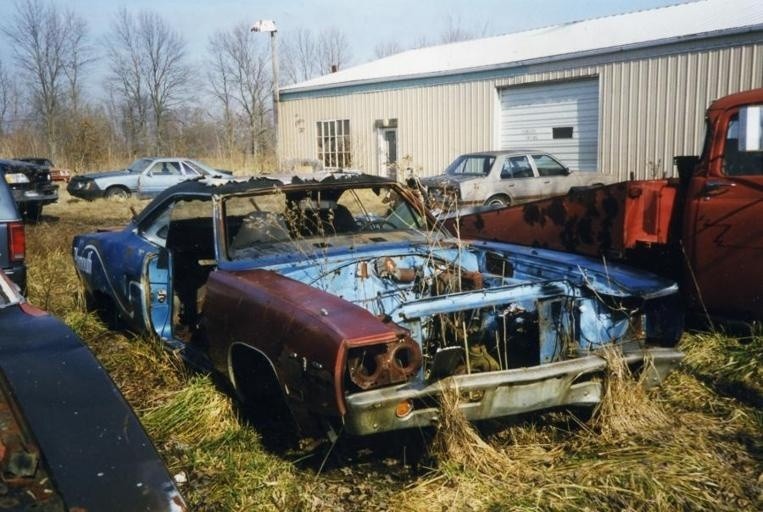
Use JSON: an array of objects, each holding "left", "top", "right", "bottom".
[{"left": 230, "top": 201, "right": 360, "bottom": 252}]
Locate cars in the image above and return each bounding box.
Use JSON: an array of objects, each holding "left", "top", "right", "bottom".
[
  {"left": 0, "top": 156, "right": 70, "bottom": 298},
  {"left": 420, "top": 150, "right": 615, "bottom": 212},
  {"left": 67, "top": 156, "right": 237, "bottom": 207}
]
